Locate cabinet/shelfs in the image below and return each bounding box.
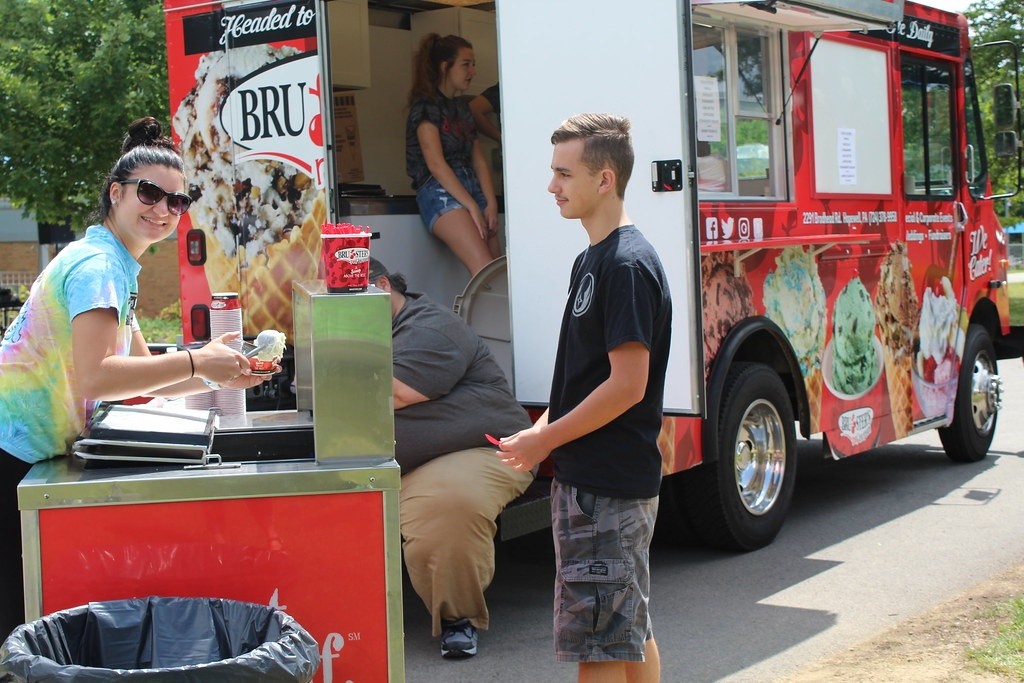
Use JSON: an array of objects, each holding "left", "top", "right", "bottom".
[
  {"left": 326, "top": 0, "right": 371, "bottom": 92},
  {"left": 410, "top": 7, "right": 503, "bottom": 198}
]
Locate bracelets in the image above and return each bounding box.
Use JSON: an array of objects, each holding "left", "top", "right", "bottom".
[
  {"left": 203, "top": 379, "right": 222, "bottom": 391},
  {"left": 183, "top": 349, "right": 195, "bottom": 379}
]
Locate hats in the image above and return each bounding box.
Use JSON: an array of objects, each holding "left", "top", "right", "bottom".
[{"left": 369, "top": 254, "right": 386, "bottom": 282}]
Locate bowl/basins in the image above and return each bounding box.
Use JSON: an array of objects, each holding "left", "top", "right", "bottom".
[
  {"left": 247, "top": 354, "right": 277, "bottom": 376},
  {"left": 184, "top": 292, "right": 247, "bottom": 417}
]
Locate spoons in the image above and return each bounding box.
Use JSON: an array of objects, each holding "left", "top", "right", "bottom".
[{"left": 485, "top": 433, "right": 502, "bottom": 445}]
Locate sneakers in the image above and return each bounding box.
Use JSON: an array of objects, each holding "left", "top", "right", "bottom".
[{"left": 440, "top": 616, "right": 478, "bottom": 657}]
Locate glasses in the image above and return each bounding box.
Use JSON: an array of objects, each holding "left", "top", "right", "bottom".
[{"left": 119, "top": 178, "right": 194, "bottom": 216}]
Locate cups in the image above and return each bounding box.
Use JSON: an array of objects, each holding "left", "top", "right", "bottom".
[
  {"left": 911, "top": 326, "right": 966, "bottom": 419},
  {"left": 320, "top": 233, "right": 372, "bottom": 293},
  {"left": 821, "top": 332, "right": 885, "bottom": 461}
]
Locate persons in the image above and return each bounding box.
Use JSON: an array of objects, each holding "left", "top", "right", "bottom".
[
  {"left": 0, "top": 115, "right": 274, "bottom": 648},
  {"left": 406, "top": 31, "right": 502, "bottom": 279},
  {"left": 496, "top": 113, "right": 672, "bottom": 683},
  {"left": 369, "top": 257, "right": 539, "bottom": 660}
]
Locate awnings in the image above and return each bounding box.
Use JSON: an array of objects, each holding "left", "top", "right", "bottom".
[
  {"left": 690, "top": 0, "right": 896, "bottom": 125},
  {"left": 692, "top": 23, "right": 771, "bottom": 117}
]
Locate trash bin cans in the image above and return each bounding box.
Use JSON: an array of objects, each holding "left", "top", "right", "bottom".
[{"left": 2, "top": 593, "right": 299, "bottom": 683}]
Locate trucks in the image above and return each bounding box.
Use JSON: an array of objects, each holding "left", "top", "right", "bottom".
[{"left": 163, "top": 0, "right": 1024, "bottom": 559}]
